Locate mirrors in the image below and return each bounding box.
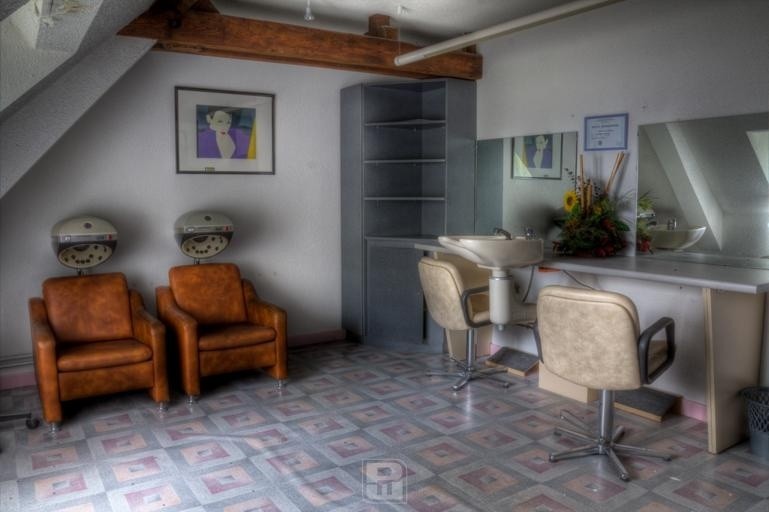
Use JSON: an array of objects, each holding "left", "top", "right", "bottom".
[{"left": 473, "top": 111, "right": 769, "bottom": 269}]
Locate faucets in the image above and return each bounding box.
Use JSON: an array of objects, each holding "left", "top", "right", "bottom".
[
  {"left": 667, "top": 217, "right": 677, "bottom": 227},
  {"left": 492, "top": 227, "right": 513, "bottom": 239}
]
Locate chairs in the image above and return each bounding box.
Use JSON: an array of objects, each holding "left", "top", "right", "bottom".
[
  {"left": 27, "top": 262, "right": 290, "bottom": 432},
  {"left": 415, "top": 256, "right": 678, "bottom": 482}
]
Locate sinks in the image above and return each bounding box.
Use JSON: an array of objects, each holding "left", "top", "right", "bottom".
[
  {"left": 438, "top": 233, "right": 544, "bottom": 268},
  {"left": 647, "top": 223, "right": 706, "bottom": 249}
]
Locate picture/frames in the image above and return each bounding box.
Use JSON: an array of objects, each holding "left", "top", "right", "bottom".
[
  {"left": 511, "top": 133, "right": 564, "bottom": 181},
  {"left": 174, "top": 85, "right": 276, "bottom": 174}
]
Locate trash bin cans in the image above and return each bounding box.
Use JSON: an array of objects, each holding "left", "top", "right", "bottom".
[{"left": 742, "top": 387, "right": 769, "bottom": 457}]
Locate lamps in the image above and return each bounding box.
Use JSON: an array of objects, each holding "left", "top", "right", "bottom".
[{"left": 304, "top": 0, "right": 315, "bottom": 22}]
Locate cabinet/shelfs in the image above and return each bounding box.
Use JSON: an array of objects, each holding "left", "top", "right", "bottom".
[{"left": 340, "top": 78, "right": 477, "bottom": 352}]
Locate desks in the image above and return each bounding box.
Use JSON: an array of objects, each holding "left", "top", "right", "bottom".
[{"left": 412, "top": 235, "right": 769, "bottom": 454}]
